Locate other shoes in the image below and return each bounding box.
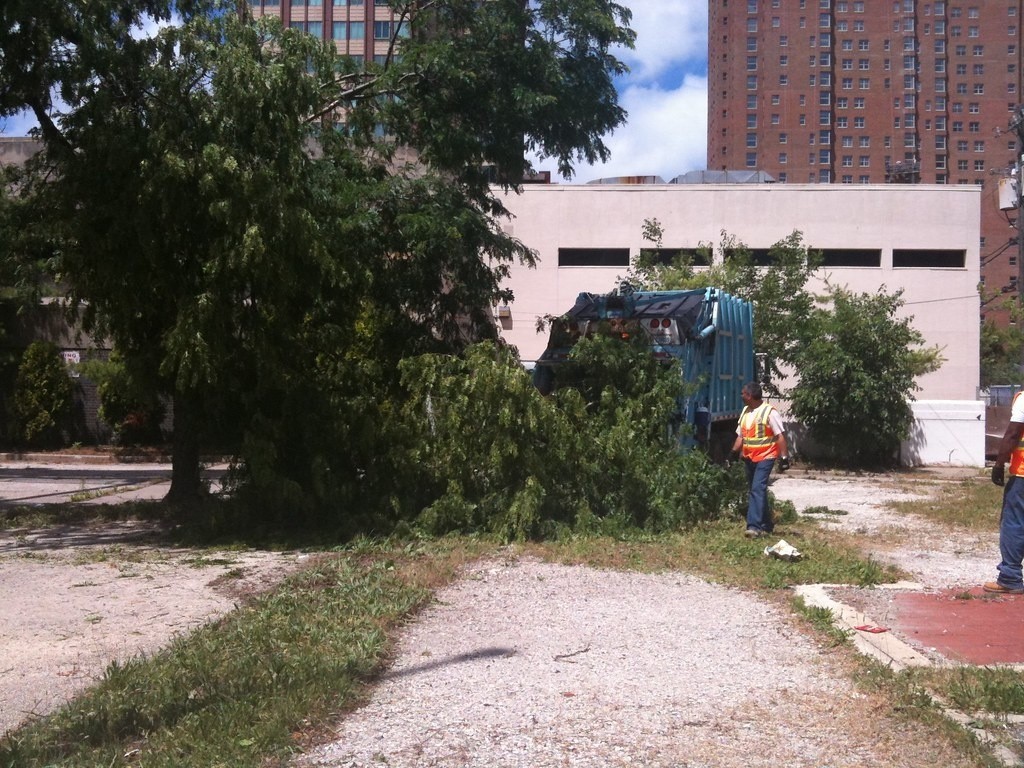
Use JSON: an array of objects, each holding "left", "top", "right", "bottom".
[
  {"left": 744, "top": 529, "right": 759, "bottom": 538},
  {"left": 759, "top": 529, "right": 773, "bottom": 537}
]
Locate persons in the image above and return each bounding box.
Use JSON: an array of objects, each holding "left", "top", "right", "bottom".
[
  {"left": 724, "top": 382, "right": 789, "bottom": 538},
  {"left": 983, "top": 379, "right": 1024, "bottom": 594}
]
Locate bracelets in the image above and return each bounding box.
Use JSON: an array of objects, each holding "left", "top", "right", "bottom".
[{"left": 781, "top": 456, "right": 787, "bottom": 460}]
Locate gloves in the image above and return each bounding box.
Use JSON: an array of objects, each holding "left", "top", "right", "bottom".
[
  {"left": 992, "top": 467, "right": 1004, "bottom": 486},
  {"left": 725, "top": 450, "right": 736, "bottom": 468},
  {"left": 778, "top": 458, "right": 790, "bottom": 471}
]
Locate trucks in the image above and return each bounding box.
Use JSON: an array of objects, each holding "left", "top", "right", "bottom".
[{"left": 531, "top": 287, "right": 754, "bottom": 467}]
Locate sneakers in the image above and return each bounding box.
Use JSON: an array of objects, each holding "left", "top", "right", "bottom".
[{"left": 984, "top": 582, "right": 1024, "bottom": 594}]
